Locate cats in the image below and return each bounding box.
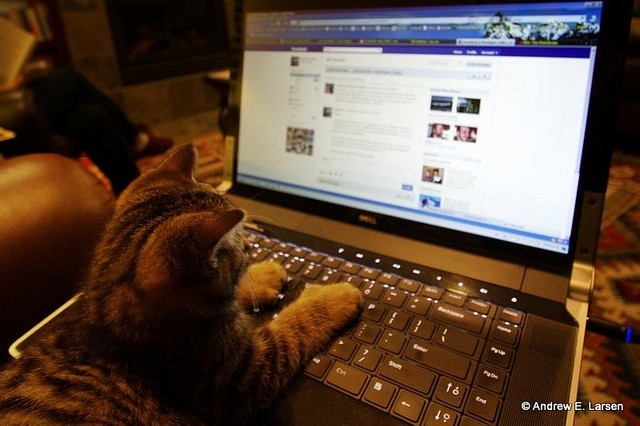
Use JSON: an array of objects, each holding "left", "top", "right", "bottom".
[{"left": 0, "top": 144, "right": 364, "bottom": 426}]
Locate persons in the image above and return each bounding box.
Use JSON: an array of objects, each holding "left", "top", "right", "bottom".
[
  {"left": 432, "top": 169, "right": 443, "bottom": 184},
  {"left": 453, "top": 124, "right": 477, "bottom": 142},
  {"left": 423, "top": 170, "right": 432, "bottom": 181},
  {"left": 1, "top": 39, "right": 174, "bottom": 200},
  {"left": 430, "top": 124, "right": 447, "bottom": 140}
]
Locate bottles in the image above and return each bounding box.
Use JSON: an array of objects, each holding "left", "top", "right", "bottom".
[{"left": 220, "top": 65, "right": 241, "bottom": 181}]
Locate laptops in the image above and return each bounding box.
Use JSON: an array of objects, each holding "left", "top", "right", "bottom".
[{"left": 8, "top": 0, "right": 631, "bottom": 426}]
